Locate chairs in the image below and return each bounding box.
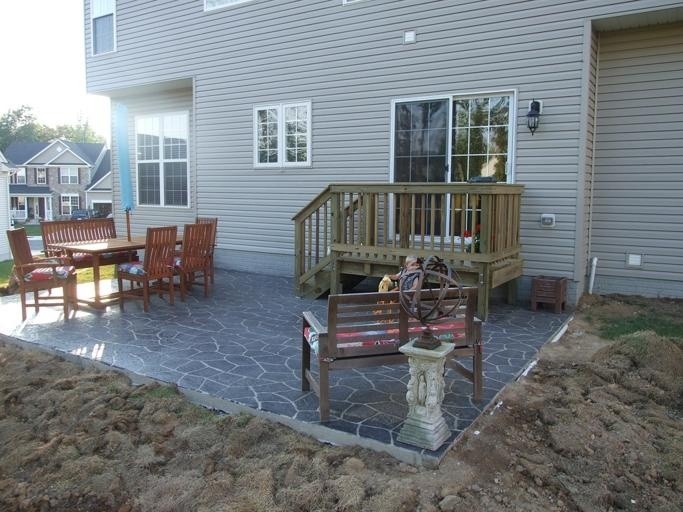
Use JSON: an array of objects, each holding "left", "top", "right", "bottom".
[
  {"left": 115, "top": 225, "right": 178, "bottom": 310},
  {"left": 186, "top": 214, "right": 218, "bottom": 283},
  {"left": 157, "top": 223, "right": 214, "bottom": 304},
  {"left": 5, "top": 227, "right": 78, "bottom": 326}
]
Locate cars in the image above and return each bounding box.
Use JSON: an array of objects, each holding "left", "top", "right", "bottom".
[{"left": 71, "top": 208, "right": 112, "bottom": 221}]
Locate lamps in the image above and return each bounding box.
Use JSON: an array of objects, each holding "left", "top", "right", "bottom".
[{"left": 524, "top": 98, "right": 542, "bottom": 135}]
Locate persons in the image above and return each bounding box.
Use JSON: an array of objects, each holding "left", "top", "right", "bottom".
[{"left": 385, "top": 254, "right": 420, "bottom": 303}]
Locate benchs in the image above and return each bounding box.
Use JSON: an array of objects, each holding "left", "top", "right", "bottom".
[
  {"left": 298, "top": 284, "right": 485, "bottom": 427},
  {"left": 38, "top": 217, "right": 141, "bottom": 297}
]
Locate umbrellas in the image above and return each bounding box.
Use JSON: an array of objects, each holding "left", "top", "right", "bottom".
[{"left": 116, "top": 102, "right": 135, "bottom": 290}]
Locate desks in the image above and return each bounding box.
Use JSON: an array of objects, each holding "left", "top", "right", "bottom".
[{"left": 46, "top": 236, "right": 188, "bottom": 316}]
